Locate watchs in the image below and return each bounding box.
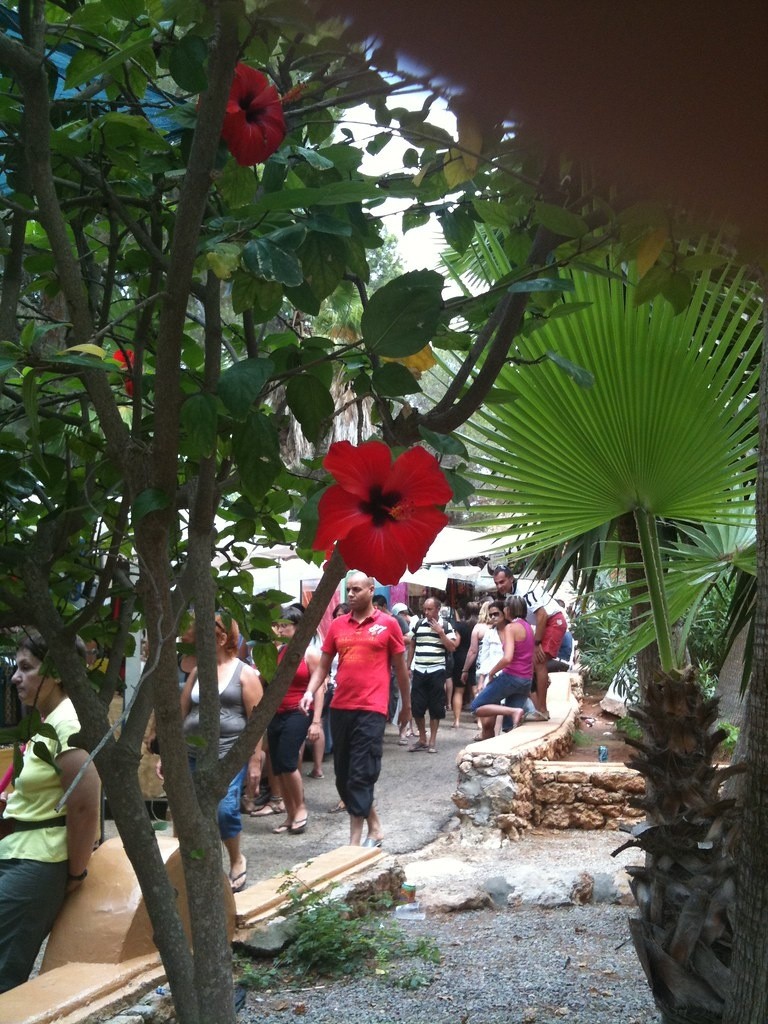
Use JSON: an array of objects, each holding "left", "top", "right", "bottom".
[
  {"left": 461, "top": 669, "right": 469, "bottom": 673},
  {"left": 67, "top": 868, "right": 88, "bottom": 881}
]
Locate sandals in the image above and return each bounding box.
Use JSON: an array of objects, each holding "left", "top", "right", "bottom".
[{"left": 251, "top": 796, "right": 287, "bottom": 817}]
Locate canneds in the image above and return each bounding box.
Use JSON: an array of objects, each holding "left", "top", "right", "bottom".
[
  {"left": 400, "top": 882, "right": 416, "bottom": 904},
  {"left": 598, "top": 745, "right": 608, "bottom": 762}
]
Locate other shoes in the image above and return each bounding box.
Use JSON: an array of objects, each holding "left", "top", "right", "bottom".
[
  {"left": 473, "top": 734, "right": 483, "bottom": 740},
  {"left": 327, "top": 805, "right": 347, "bottom": 813},
  {"left": 399, "top": 728, "right": 409, "bottom": 745},
  {"left": 525, "top": 710, "right": 549, "bottom": 721}
]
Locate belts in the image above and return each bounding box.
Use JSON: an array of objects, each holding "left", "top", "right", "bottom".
[{"left": 552, "top": 656, "right": 570, "bottom": 665}]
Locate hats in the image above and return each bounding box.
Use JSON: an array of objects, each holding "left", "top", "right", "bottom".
[{"left": 391, "top": 603, "right": 409, "bottom": 615}]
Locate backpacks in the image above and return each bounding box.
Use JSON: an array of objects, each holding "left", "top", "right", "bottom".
[{"left": 414, "top": 619, "right": 456, "bottom": 678}]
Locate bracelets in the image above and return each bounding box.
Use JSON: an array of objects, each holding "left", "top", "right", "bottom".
[{"left": 312, "top": 721, "right": 322, "bottom": 726}]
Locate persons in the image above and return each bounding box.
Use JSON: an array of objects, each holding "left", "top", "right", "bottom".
[{"left": 1, "top": 565, "right": 572, "bottom": 996}]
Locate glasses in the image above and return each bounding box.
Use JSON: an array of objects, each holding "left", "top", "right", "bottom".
[
  {"left": 86, "top": 648, "right": 98, "bottom": 657},
  {"left": 489, "top": 612, "right": 499, "bottom": 618}
]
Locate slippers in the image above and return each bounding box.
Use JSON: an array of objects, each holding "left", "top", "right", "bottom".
[
  {"left": 273, "top": 824, "right": 291, "bottom": 834},
  {"left": 512, "top": 712, "right": 529, "bottom": 729},
  {"left": 362, "top": 838, "right": 384, "bottom": 848},
  {"left": 228, "top": 871, "right": 248, "bottom": 893},
  {"left": 290, "top": 811, "right": 309, "bottom": 834},
  {"left": 407, "top": 741, "right": 429, "bottom": 751},
  {"left": 428, "top": 743, "right": 438, "bottom": 753},
  {"left": 306, "top": 771, "right": 325, "bottom": 779}
]
